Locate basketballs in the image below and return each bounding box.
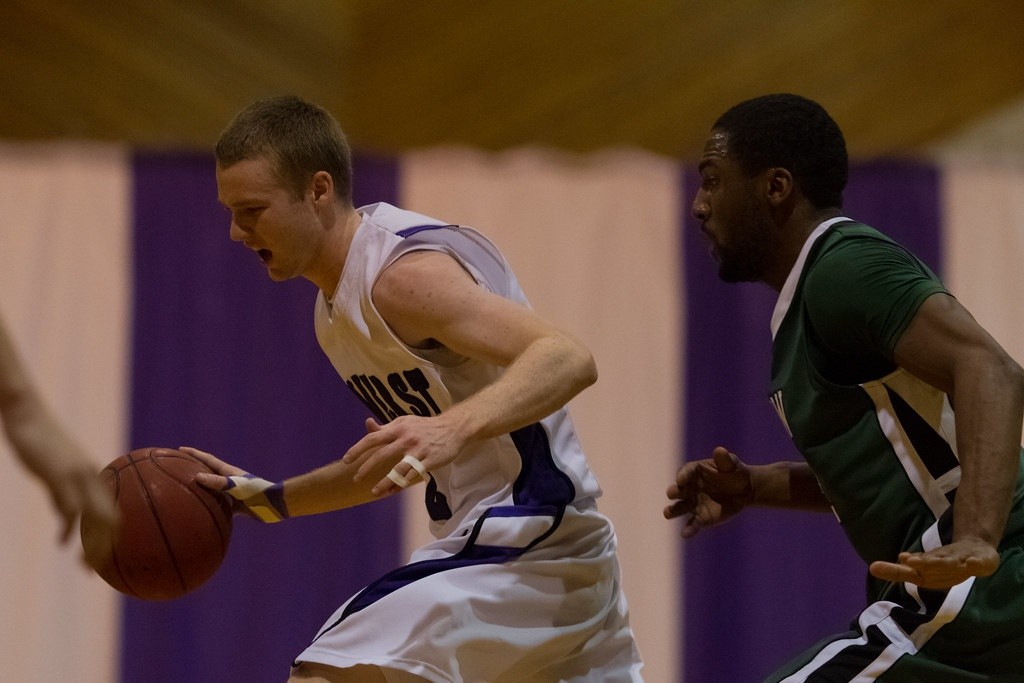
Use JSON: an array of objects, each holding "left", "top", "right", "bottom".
[{"left": 78, "top": 444, "right": 235, "bottom": 604}]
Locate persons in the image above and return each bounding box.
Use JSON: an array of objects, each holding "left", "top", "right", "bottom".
[
  {"left": 179, "top": 95, "right": 646, "bottom": 683},
  {"left": 0, "top": 316, "right": 127, "bottom": 555},
  {"left": 662, "top": 93, "right": 1024, "bottom": 683}
]
